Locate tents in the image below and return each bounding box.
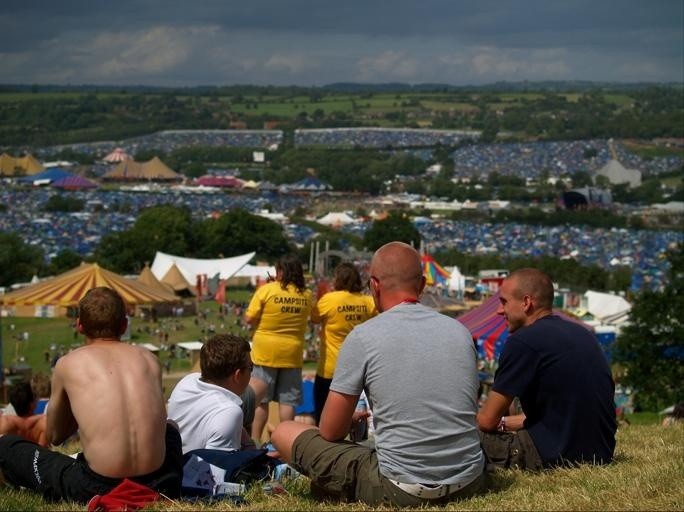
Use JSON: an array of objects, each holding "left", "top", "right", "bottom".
[
  {"left": 0, "top": 262, "right": 196, "bottom": 317},
  {"left": 0, "top": 152, "right": 45, "bottom": 176}
]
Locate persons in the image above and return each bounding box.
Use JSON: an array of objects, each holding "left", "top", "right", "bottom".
[
  {"left": 0, "top": 382, "right": 49, "bottom": 447},
  {"left": 0, "top": 287, "right": 183, "bottom": 504},
  {"left": 311, "top": 262, "right": 379, "bottom": 429},
  {"left": 270, "top": 240, "right": 484, "bottom": 505},
  {"left": 166, "top": 336, "right": 270, "bottom": 481},
  {"left": 243, "top": 255, "right": 312, "bottom": 441},
  {"left": 476, "top": 268, "right": 616, "bottom": 470}
]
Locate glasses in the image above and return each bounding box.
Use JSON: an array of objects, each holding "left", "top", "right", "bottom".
[
  {"left": 367, "top": 276, "right": 379, "bottom": 290},
  {"left": 239, "top": 364, "right": 254, "bottom": 372}
]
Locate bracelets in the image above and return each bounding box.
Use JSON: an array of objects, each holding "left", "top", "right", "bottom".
[
  {"left": 242, "top": 441, "right": 255, "bottom": 446},
  {"left": 501, "top": 416, "right": 505, "bottom": 431}
]
